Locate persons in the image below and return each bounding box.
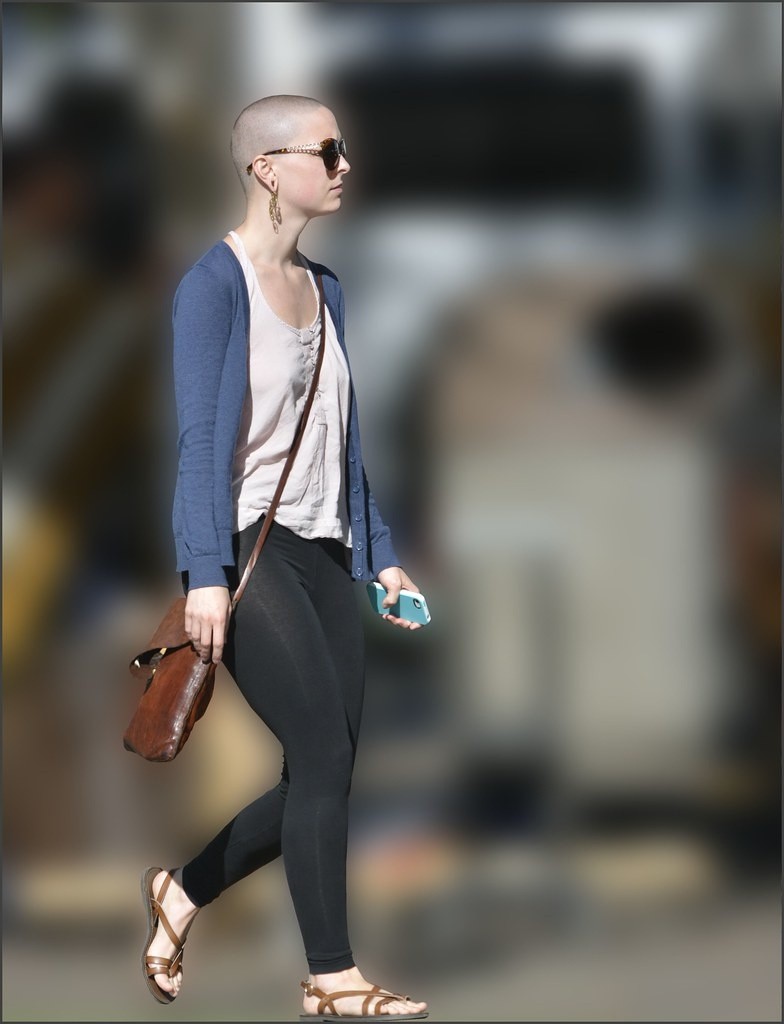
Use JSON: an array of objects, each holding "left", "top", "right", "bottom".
[{"left": 141, "top": 96, "right": 430, "bottom": 1019}]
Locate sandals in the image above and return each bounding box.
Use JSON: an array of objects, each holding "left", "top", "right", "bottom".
[
  {"left": 299, "top": 980, "right": 429, "bottom": 1024},
  {"left": 140, "top": 866, "right": 188, "bottom": 1004}
]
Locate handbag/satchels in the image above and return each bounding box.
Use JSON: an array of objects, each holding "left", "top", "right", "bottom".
[{"left": 122, "top": 597, "right": 232, "bottom": 762}]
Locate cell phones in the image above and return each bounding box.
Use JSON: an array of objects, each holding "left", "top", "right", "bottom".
[{"left": 367, "top": 582, "right": 431, "bottom": 625}]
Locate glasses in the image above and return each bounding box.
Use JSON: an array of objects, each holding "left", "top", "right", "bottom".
[{"left": 246, "top": 137, "right": 347, "bottom": 175}]
